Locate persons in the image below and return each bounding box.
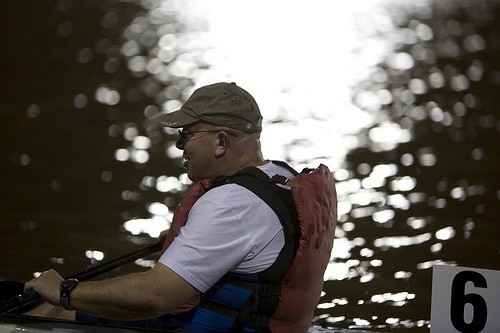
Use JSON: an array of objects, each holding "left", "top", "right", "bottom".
[{"left": 24, "top": 81, "right": 301, "bottom": 333}]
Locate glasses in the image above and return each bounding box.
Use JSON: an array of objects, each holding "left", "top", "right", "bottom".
[{"left": 177, "top": 129, "right": 238, "bottom": 145}]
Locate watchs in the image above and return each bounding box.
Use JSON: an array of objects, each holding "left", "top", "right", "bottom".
[{"left": 60, "top": 278, "right": 79, "bottom": 311}]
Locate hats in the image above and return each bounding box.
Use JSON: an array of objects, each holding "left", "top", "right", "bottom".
[{"left": 152, "top": 82, "right": 263, "bottom": 133}]
